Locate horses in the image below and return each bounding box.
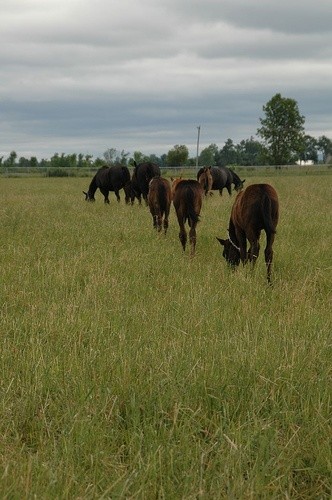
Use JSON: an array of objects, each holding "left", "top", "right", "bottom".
[
  {"left": 148, "top": 176, "right": 203, "bottom": 258},
  {"left": 81, "top": 160, "right": 161, "bottom": 207},
  {"left": 215, "top": 183, "right": 279, "bottom": 287},
  {"left": 197, "top": 165, "right": 246, "bottom": 197}
]
